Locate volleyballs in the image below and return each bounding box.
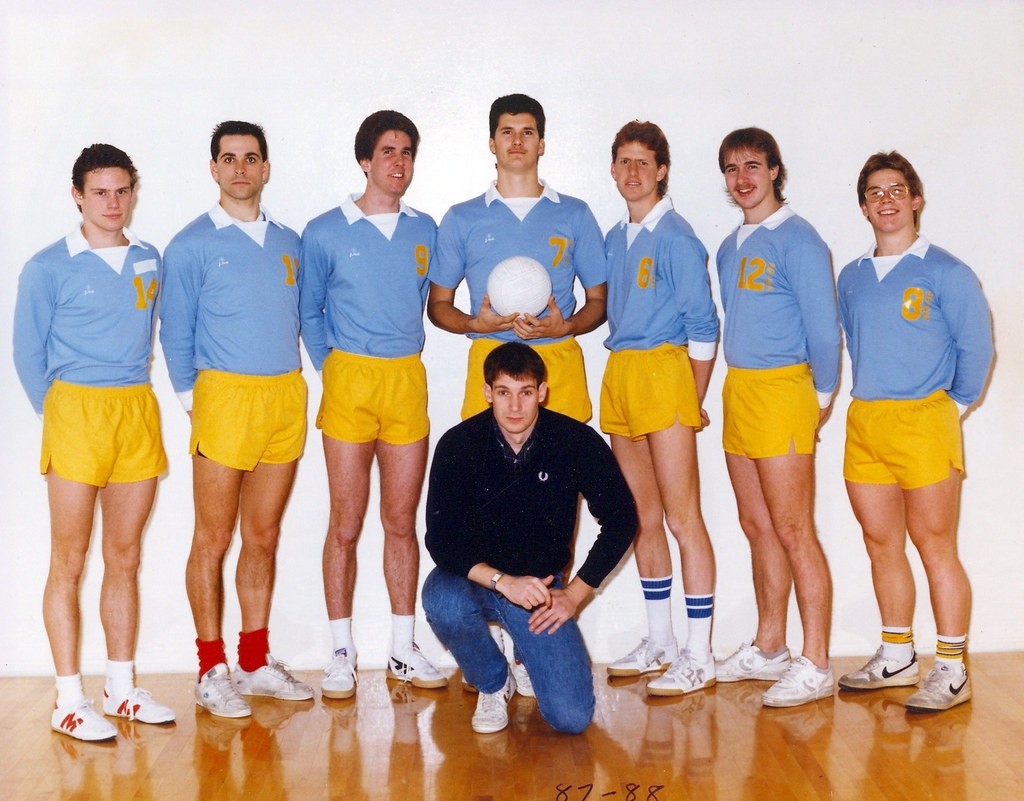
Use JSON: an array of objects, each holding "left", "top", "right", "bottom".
[{"left": 486, "top": 256, "right": 552, "bottom": 319}]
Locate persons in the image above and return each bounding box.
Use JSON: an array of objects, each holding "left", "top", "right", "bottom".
[
  {"left": 419, "top": 342, "right": 642, "bottom": 734},
  {"left": 600, "top": 121, "right": 720, "bottom": 696},
  {"left": 717, "top": 127, "right": 841, "bottom": 706},
  {"left": 158, "top": 120, "right": 313, "bottom": 718},
  {"left": 836, "top": 151, "right": 996, "bottom": 711},
  {"left": 426, "top": 95, "right": 607, "bottom": 696},
  {"left": 297, "top": 111, "right": 446, "bottom": 699},
  {"left": 12, "top": 144, "right": 175, "bottom": 741}
]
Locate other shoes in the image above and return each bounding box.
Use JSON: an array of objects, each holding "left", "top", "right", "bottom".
[
  {"left": 512, "top": 653, "right": 535, "bottom": 697},
  {"left": 460, "top": 630, "right": 505, "bottom": 692}
]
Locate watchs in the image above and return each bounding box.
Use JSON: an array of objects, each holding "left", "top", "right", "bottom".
[{"left": 490, "top": 571, "right": 505, "bottom": 591}]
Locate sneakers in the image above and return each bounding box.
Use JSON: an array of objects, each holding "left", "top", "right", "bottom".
[
  {"left": 472, "top": 667, "right": 517, "bottom": 734},
  {"left": 607, "top": 638, "right": 679, "bottom": 679},
  {"left": 727, "top": 684, "right": 770, "bottom": 713},
  {"left": 324, "top": 699, "right": 361, "bottom": 728},
  {"left": 233, "top": 653, "right": 316, "bottom": 702},
  {"left": 249, "top": 700, "right": 316, "bottom": 730},
  {"left": 102, "top": 685, "right": 177, "bottom": 724},
  {"left": 391, "top": 680, "right": 436, "bottom": 711},
  {"left": 715, "top": 642, "right": 792, "bottom": 683},
  {"left": 55, "top": 740, "right": 117, "bottom": 763},
  {"left": 663, "top": 688, "right": 721, "bottom": 720},
  {"left": 116, "top": 720, "right": 176, "bottom": 744},
  {"left": 195, "top": 662, "right": 253, "bottom": 718},
  {"left": 645, "top": 649, "right": 716, "bottom": 697},
  {"left": 386, "top": 643, "right": 448, "bottom": 690},
  {"left": 50, "top": 703, "right": 120, "bottom": 741},
  {"left": 839, "top": 685, "right": 920, "bottom": 719},
  {"left": 905, "top": 708, "right": 973, "bottom": 740},
  {"left": 195, "top": 713, "right": 252, "bottom": 751},
  {"left": 904, "top": 658, "right": 973, "bottom": 712},
  {"left": 761, "top": 656, "right": 834, "bottom": 707},
  {"left": 774, "top": 711, "right": 830, "bottom": 740},
  {"left": 322, "top": 648, "right": 358, "bottom": 700},
  {"left": 837, "top": 641, "right": 918, "bottom": 690}
]
